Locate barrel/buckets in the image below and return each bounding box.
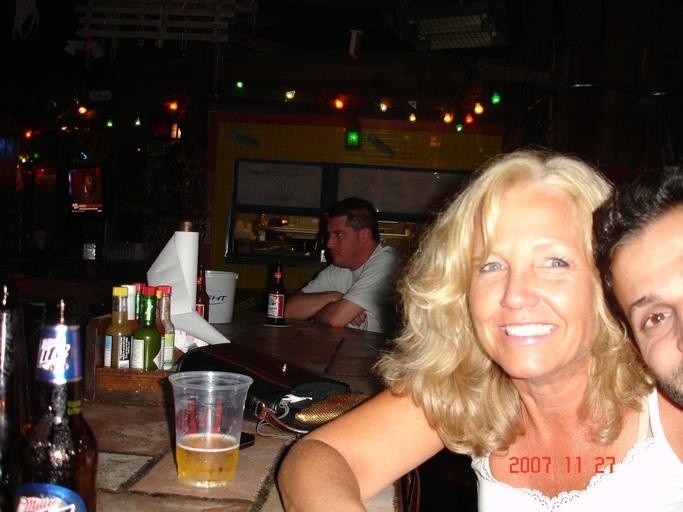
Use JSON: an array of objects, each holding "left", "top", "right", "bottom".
[{"left": 203, "top": 271, "right": 239, "bottom": 325}]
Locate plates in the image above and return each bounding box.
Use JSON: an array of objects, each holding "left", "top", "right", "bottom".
[
  {"left": 271, "top": 226, "right": 322, "bottom": 239},
  {"left": 379, "top": 233, "right": 408, "bottom": 242}
]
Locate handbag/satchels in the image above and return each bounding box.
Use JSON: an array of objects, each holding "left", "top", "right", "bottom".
[{"left": 178, "top": 344, "right": 352, "bottom": 436}]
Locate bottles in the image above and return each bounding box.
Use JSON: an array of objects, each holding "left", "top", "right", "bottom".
[
  {"left": 195, "top": 264, "right": 209, "bottom": 323},
  {"left": 1, "top": 284, "right": 174, "bottom": 512},
  {"left": 264, "top": 262, "right": 285, "bottom": 325}
]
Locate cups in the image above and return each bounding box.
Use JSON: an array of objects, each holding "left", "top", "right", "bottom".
[{"left": 169, "top": 372, "right": 253, "bottom": 485}]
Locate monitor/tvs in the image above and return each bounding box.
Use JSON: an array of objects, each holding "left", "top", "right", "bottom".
[{"left": 65, "top": 161, "right": 110, "bottom": 217}]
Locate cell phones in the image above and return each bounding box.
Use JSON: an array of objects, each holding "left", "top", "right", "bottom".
[{"left": 238, "top": 432, "right": 255, "bottom": 450}]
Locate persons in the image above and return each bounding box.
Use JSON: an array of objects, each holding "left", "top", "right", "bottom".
[
  {"left": 590, "top": 163, "right": 683, "bottom": 410},
  {"left": 280, "top": 195, "right": 400, "bottom": 333},
  {"left": 271, "top": 143, "right": 683, "bottom": 510}
]
